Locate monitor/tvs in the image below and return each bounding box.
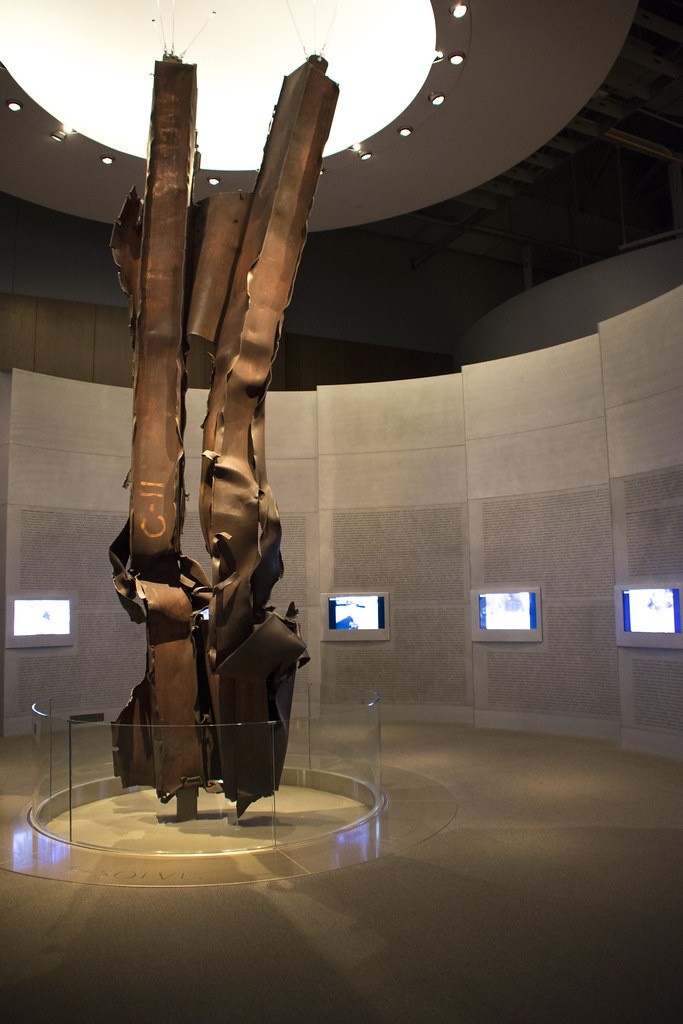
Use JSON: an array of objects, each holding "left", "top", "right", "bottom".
[
  {"left": 199, "top": 608, "right": 210, "bottom": 621},
  {"left": 470, "top": 586, "right": 543, "bottom": 642},
  {"left": 614, "top": 582, "right": 683, "bottom": 649},
  {"left": 320, "top": 592, "right": 389, "bottom": 642},
  {"left": 5, "top": 594, "right": 74, "bottom": 649}
]
